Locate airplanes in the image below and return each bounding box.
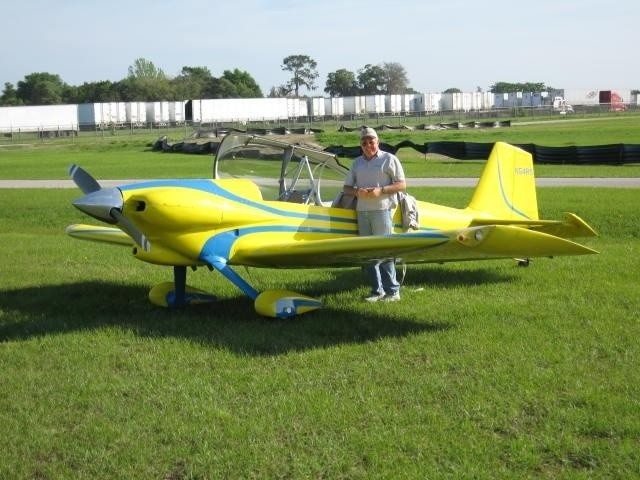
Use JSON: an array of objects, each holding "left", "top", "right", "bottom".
[{"left": 60, "top": 128, "right": 615, "bottom": 322}]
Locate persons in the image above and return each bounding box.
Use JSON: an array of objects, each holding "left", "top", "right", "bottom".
[{"left": 343, "top": 128, "right": 406, "bottom": 302}]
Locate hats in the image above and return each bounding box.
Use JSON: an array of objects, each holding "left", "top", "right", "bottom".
[{"left": 360, "top": 127, "right": 379, "bottom": 140}]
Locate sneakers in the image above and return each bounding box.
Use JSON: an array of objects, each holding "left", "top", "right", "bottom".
[
  {"left": 380, "top": 293, "right": 401, "bottom": 302},
  {"left": 364, "top": 293, "right": 386, "bottom": 302}
]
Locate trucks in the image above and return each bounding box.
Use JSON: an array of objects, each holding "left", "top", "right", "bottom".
[
  {"left": 586, "top": 91, "right": 628, "bottom": 113},
  {"left": 555, "top": 105, "right": 575, "bottom": 114}
]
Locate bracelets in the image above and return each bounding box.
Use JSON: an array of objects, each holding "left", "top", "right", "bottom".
[{"left": 381, "top": 185, "right": 385, "bottom": 194}]
